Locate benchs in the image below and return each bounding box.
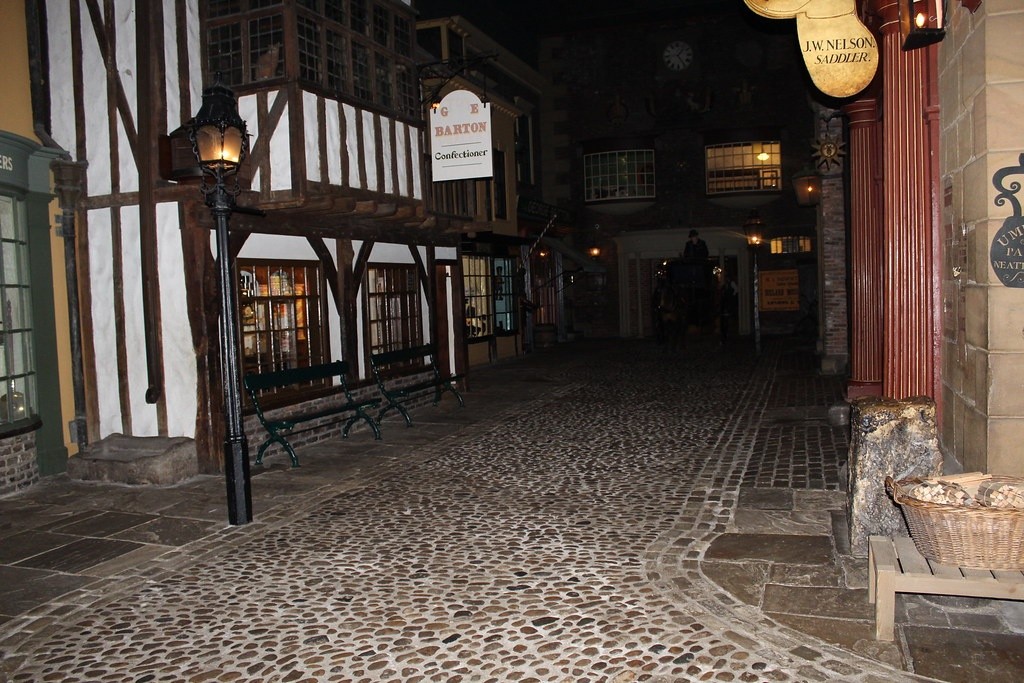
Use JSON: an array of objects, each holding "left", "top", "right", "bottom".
[
  {"left": 244, "top": 359, "right": 382, "bottom": 467},
  {"left": 370, "top": 344, "right": 465, "bottom": 428}
]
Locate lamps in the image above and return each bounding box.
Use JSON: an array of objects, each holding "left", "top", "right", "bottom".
[
  {"left": 899, "top": 1, "right": 946, "bottom": 52},
  {"left": 790, "top": 166, "right": 825, "bottom": 207}
]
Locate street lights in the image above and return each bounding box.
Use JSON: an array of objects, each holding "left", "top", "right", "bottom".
[
  {"left": 589, "top": 233, "right": 610, "bottom": 344},
  {"left": 744, "top": 216, "right": 766, "bottom": 355},
  {"left": 189, "top": 85, "right": 255, "bottom": 526}
]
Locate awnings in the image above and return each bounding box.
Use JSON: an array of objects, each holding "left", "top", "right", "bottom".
[{"left": 531, "top": 236, "right": 609, "bottom": 295}]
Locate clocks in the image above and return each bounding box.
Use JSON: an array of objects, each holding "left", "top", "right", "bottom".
[{"left": 661, "top": 39, "right": 697, "bottom": 76}]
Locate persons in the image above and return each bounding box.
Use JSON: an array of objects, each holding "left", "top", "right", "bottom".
[
  {"left": 683, "top": 229, "right": 709, "bottom": 260},
  {"left": 721, "top": 274, "right": 739, "bottom": 312}
]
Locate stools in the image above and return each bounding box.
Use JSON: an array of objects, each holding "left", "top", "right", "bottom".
[{"left": 866, "top": 535, "right": 1024, "bottom": 644}]
